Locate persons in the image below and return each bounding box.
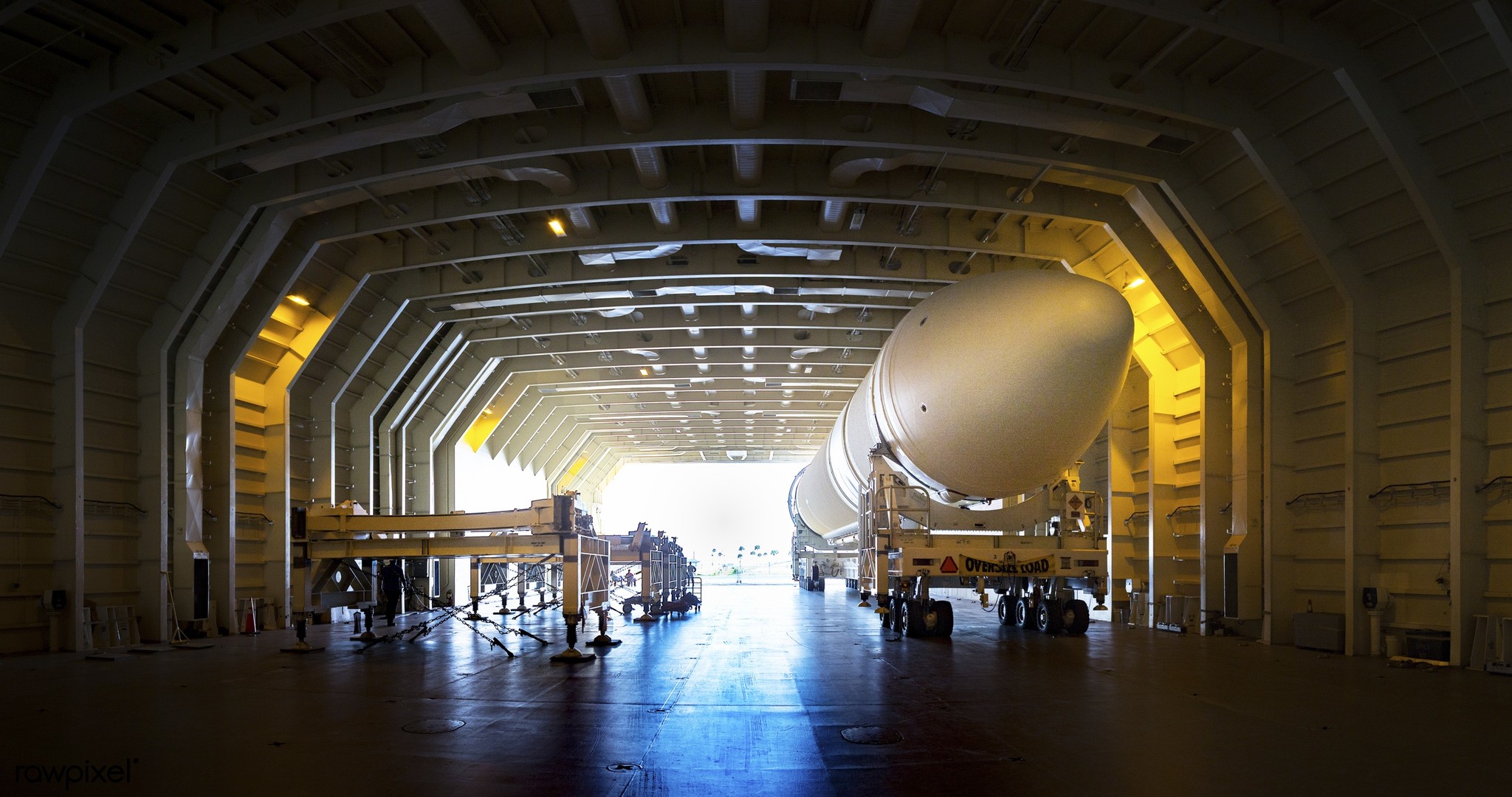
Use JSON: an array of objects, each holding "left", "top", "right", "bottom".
[
  {"left": 611, "top": 571, "right": 625, "bottom": 582},
  {"left": 377, "top": 560, "right": 407, "bottom": 626},
  {"left": 687, "top": 561, "right": 696, "bottom": 586},
  {"left": 625, "top": 569, "right": 636, "bottom": 587}
]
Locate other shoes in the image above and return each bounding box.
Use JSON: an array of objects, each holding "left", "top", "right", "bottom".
[{"left": 387, "top": 621, "right": 397, "bottom": 626}]
